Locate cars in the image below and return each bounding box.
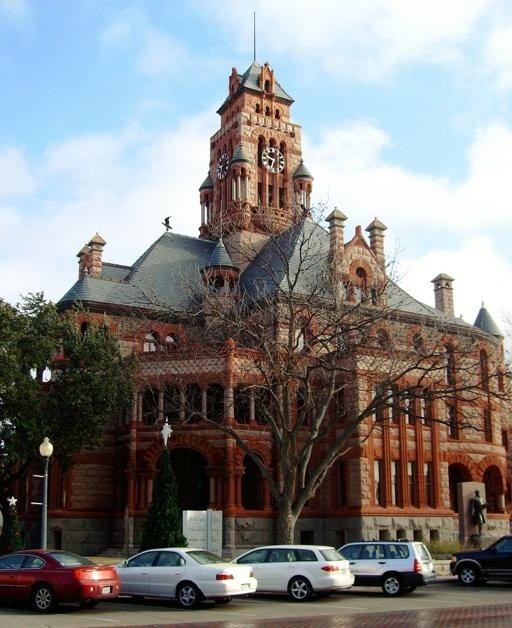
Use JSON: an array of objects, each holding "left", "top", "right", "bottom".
[
  {"left": 0, "top": 549, "right": 121, "bottom": 613},
  {"left": 229, "top": 544, "right": 354, "bottom": 601},
  {"left": 449, "top": 534, "right": 512, "bottom": 586},
  {"left": 110, "top": 547, "right": 258, "bottom": 609}
]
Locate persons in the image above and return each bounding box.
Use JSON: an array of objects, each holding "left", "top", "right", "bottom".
[{"left": 471, "top": 488, "right": 487, "bottom": 536}]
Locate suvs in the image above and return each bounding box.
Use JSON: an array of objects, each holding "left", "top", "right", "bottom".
[{"left": 337, "top": 538, "right": 438, "bottom": 597}]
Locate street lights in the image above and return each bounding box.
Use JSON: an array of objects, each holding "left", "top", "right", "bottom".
[{"left": 39, "top": 436, "right": 55, "bottom": 549}]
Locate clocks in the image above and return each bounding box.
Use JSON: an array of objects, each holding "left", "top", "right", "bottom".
[
  {"left": 215, "top": 152, "right": 231, "bottom": 180},
  {"left": 261, "top": 147, "right": 286, "bottom": 175}
]
